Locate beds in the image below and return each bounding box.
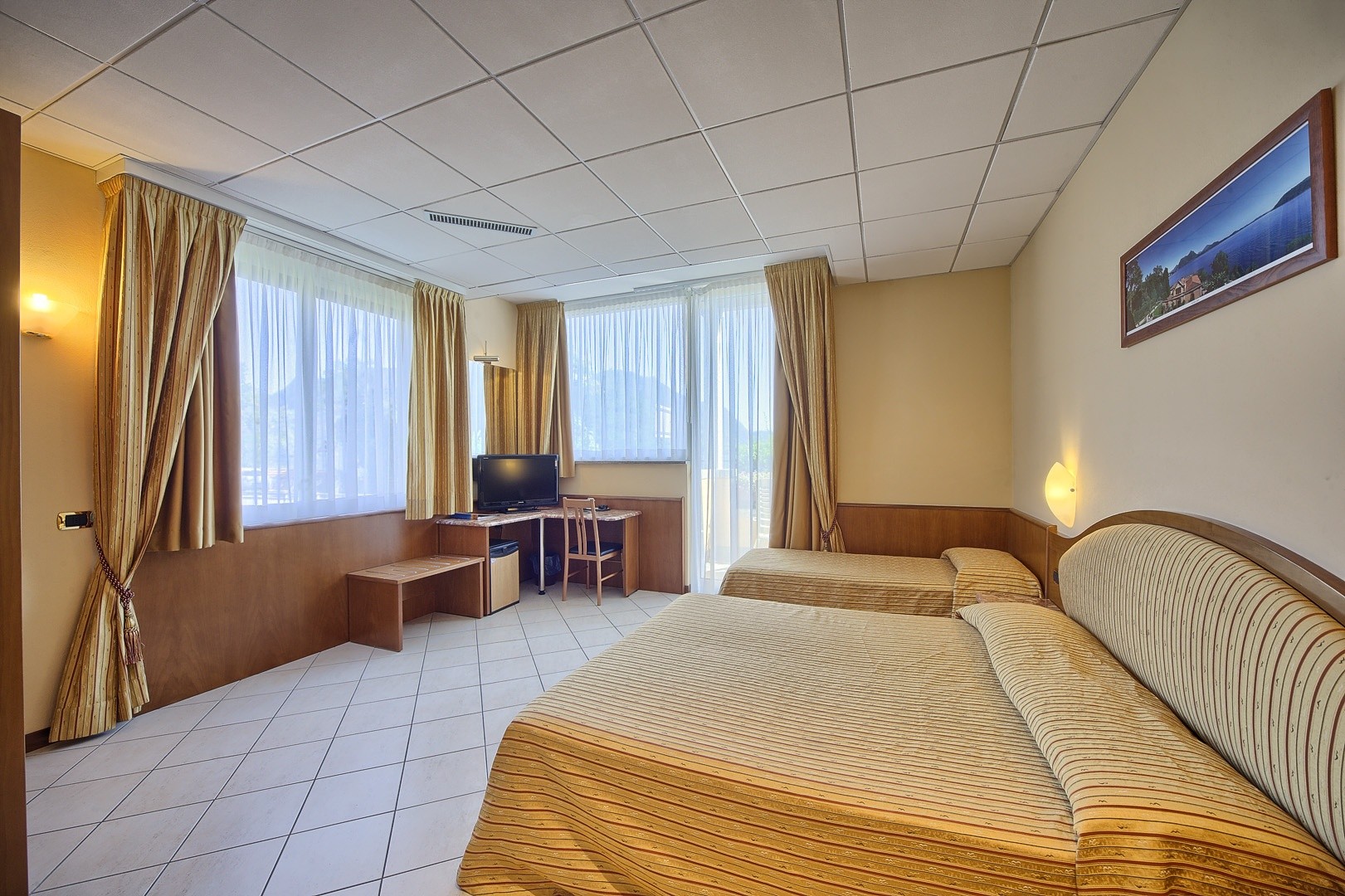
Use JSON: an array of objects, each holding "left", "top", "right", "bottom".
[
  {"left": 720, "top": 548, "right": 1043, "bottom": 618},
  {"left": 455, "top": 509, "right": 1345, "bottom": 896}
]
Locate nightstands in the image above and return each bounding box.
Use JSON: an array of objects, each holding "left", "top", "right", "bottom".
[{"left": 975, "top": 592, "right": 1062, "bottom": 612}]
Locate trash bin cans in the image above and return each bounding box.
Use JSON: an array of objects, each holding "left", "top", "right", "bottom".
[{"left": 530, "top": 551, "right": 559, "bottom": 586}]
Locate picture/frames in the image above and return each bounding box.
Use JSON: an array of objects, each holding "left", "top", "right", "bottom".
[{"left": 1121, "top": 89, "right": 1338, "bottom": 348}]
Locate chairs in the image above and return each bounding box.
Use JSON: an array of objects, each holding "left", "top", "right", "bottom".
[{"left": 562, "top": 497, "right": 623, "bottom": 606}]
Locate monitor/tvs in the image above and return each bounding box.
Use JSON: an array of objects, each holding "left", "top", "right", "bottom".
[{"left": 478, "top": 454, "right": 559, "bottom": 513}]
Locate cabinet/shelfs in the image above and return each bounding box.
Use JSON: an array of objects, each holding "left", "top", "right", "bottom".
[{"left": 489, "top": 539, "right": 520, "bottom": 615}]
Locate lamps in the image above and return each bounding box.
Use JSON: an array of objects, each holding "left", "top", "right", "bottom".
[
  {"left": 1044, "top": 462, "right": 1076, "bottom": 528},
  {"left": 22, "top": 329, "right": 53, "bottom": 341},
  {"left": 474, "top": 340, "right": 500, "bottom": 361}
]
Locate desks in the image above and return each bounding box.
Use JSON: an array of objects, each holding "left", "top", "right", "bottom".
[
  {"left": 437, "top": 507, "right": 641, "bottom": 618},
  {"left": 346, "top": 554, "right": 485, "bottom": 652}
]
